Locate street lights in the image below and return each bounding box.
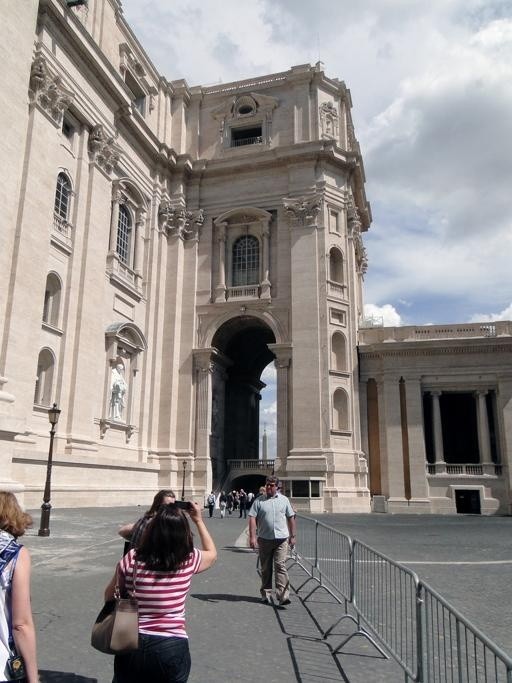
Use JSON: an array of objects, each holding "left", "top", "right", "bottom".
[
  {"left": 38, "top": 403, "right": 61, "bottom": 536},
  {"left": 181, "top": 460, "right": 188, "bottom": 502}
]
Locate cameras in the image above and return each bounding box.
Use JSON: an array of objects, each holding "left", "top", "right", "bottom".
[{"left": 173, "top": 500, "right": 192, "bottom": 512}]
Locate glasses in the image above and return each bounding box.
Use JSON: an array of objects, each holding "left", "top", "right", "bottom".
[{"left": 265, "top": 484, "right": 277, "bottom": 488}]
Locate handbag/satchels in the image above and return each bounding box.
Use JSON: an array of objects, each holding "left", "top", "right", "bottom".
[{"left": 91, "top": 599, "right": 139, "bottom": 655}]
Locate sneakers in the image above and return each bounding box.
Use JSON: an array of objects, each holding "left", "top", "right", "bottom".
[{"left": 261, "top": 593, "right": 291, "bottom": 606}]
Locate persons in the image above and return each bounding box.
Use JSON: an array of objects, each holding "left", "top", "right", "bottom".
[
  {"left": 117, "top": 489, "right": 176, "bottom": 552},
  {"left": 110, "top": 362, "right": 129, "bottom": 420},
  {"left": 103, "top": 499, "right": 218, "bottom": 683},
  {"left": 207, "top": 482, "right": 267, "bottom": 520},
  {"left": 0, "top": 489, "right": 42, "bottom": 683},
  {"left": 247, "top": 474, "right": 297, "bottom": 605}
]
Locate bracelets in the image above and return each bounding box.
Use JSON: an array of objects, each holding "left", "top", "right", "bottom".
[{"left": 289, "top": 534, "right": 296, "bottom": 538}]
